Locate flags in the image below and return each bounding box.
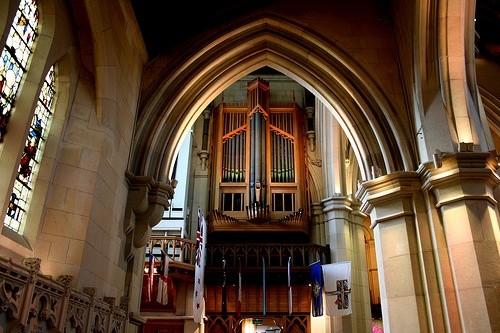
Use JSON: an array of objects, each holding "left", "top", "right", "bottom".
[
  {"left": 321, "top": 260, "right": 352, "bottom": 317},
  {"left": 222, "top": 260, "right": 227, "bottom": 321},
  {"left": 310, "top": 261, "right": 323, "bottom": 317},
  {"left": 192, "top": 208, "right": 205, "bottom": 325},
  {"left": 236, "top": 261, "right": 242, "bottom": 319},
  {"left": 156, "top": 250, "right": 170, "bottom": 306},
  {"left": 288, "top": 258, "right": 293, "bottom": 316},
  {"left": 146, "top": 252, "right": 155, "bottom": 304},
  {"left": 263, "top": 258, "right": 266, "bottom": 315}
]
